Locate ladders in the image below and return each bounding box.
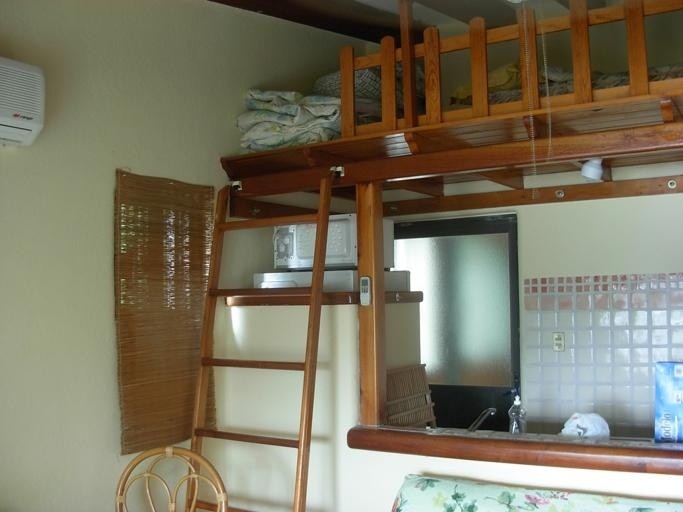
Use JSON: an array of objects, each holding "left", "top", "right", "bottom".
[{"left": 182, "top": 175, "right": 336, "bottom": 512}]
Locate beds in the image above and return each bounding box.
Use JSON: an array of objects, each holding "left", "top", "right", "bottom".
[{"left": 219, "top": 0, "right": 683, "bottom": 220}]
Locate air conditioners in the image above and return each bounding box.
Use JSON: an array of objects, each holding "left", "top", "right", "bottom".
[{"left": 0, "top": 54, "right": 46, "bottom": 148}]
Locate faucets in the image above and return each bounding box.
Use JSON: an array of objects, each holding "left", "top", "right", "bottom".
[{"left": 467, "top": 407, "right": 497, "bottom": 432}]
